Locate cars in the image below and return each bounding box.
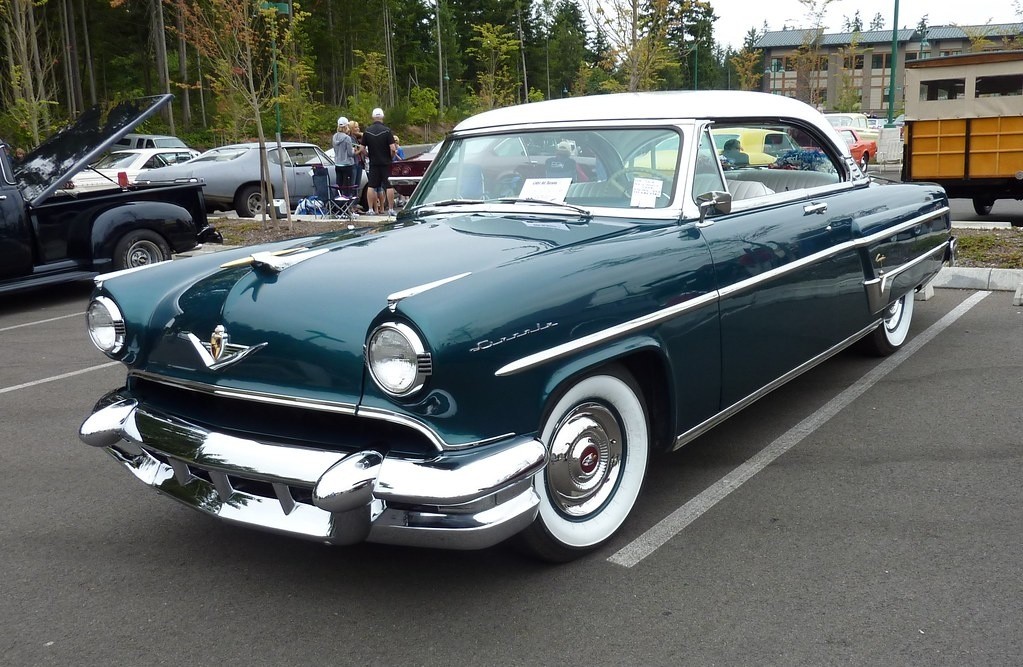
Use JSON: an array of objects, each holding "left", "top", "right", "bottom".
[
  {"left": 625, "top": 127, "right": 793, "bottom": 169},
  {"left": 389, "top": 133, "right": 600, "bottom": 199},
  {"left": 133, "top": 140, "right": 390, "bottom": 218},
  {"left": 783, "top": 127, "right": 877, "bottom": 171},
  {"left": 824, "top": 113, "right": 880, "bottom": 140},
  {"left": 868, "top": 115, "right": 904, "bottom": 129},
  {"left": 76, "top": 96, "right": 957, "bottom": 563}
]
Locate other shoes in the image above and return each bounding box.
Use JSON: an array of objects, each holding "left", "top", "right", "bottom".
[
  {"left": 388, "top": 211, "right": 397, "bottom": 216},
  {"left": 397, "top": 200, "right": 403, "bottom": 207},
  {"left": 335, "top": 214, "right": 342, "bottom": 219},
  {"left": 356, "top": 209, "right": 366, "bottom": 215},
  {"left": 343, "top": 212, "right": 359, "bottom": 219},
  {"left": 404, "top": 200, "right": 408, "bottom": 205},
  {"left": 366, "top": 211, "right": 374, "bottom": 216}
]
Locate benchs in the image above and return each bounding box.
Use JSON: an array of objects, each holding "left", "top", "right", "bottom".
[
  {"left": 565, "top": 174, "right": 775, "bottom": 202},
  {"left": 706, "top": 169, "right": 843, "bottom": 193}
]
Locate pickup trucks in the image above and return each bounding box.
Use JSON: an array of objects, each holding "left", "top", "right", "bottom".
[{"left": 0, "top": 94, "right": 227, "bottom": 294}]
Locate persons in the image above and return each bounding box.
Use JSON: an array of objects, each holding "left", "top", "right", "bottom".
[
  {"left": 373, "top": 186, "right": 386, "bottom": 215},
  {"left": 359, "top": 107, "right": 397, "bottom": 216},
  {"left": 718, "top": 138, "right": 744, "bottom": 173},
  {"left": 544, "top": 141, "right": 587, "bottom": 185},
  {"left": 332, "top": 116, "right": 364, "bottom": 218},
  {"left": 293, "top": 151, "right": 305, "bottom": 165},
  {"left": 392, "top": 135, "right": 411, "bottom": 207},
  {"left": 348, "top": 120, "right": 367, "bottom": 215},
  {"left": 13, "top": 148, "right": 26, "bottom": 165},
  {"left": 4, "top": 143, "right": 13, "bottom": 165}
]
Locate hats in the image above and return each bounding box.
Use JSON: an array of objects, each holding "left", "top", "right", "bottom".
[
  {"left": 556, "top": 141, "right": 570, "bottom": 150},
  {"left": 338, "top": 117, "right": 350, "bottom": 127},
  {"left": 372, "top": 108, "right": 384, "bottom": 117},
  {"left": 723, "top": 139, "right": 744, "bottom": 151}
]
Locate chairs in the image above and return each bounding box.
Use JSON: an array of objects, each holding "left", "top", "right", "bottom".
[{"left": 310, "top": 166, "right": 358, "bottom": 220}]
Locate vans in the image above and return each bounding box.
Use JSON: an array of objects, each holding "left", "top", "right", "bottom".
[{"left": 111, "top": 133, "right": 191, "bottom": 162}]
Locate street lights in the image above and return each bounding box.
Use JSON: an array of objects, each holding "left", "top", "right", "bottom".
[
  {"left": 765, "top": 61, "right": 787, "bottom": 95},
  {"left": 253, "top": 1, "right": 289, "bottom": 134},
  {"left": 689, "top": 44, "right": 698, "bottom": 90},
  {"left": 886, "top": 29, "right": 928, "bottom": 126}
]
